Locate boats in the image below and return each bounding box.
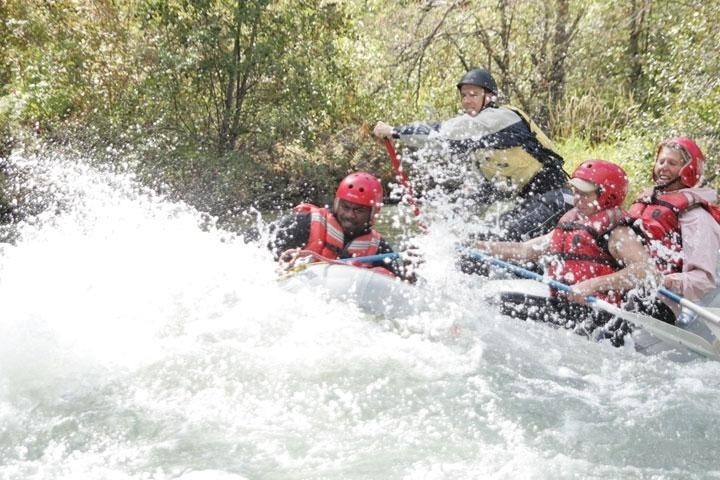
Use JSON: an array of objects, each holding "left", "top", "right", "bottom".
[{"left": 290, "top": 264, "right": 640, "bottom": 375}]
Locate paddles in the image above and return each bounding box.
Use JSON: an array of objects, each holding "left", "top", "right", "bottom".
[
  {"left": 335, "top": 227, "right": 553, "bottom": 267},
  {"left": 479, "top": 278, "right": 551, "bottom": 299},
  {"left": 458, "top": 243, "right": 720, "bottom": 362}
]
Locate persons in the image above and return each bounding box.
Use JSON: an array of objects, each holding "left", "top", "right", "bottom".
[
  {"left": 264, "top": 170, "right": 419, "bottom": 286},
  {"left": 449, "top": 155, "right": 677, "bottom": 349},
  {"left": 625, "top": 136, "right": 720, "bottom": 326},
  {"left": 373, "top": 68, "right": 577, "bottom": 282}
]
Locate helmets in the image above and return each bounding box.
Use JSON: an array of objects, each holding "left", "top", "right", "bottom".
[
  {"left": 652, "top": 137, "right": 705, "bottom": 188},
  {"left": 333, "top": 172, "right": 383, "bottom": 226},
  {"left": 572, "top": 159, "right": 629, "bottom": 211},
  {"left": 456, "top": 69, "right": 498, "bottom": 96}
]
